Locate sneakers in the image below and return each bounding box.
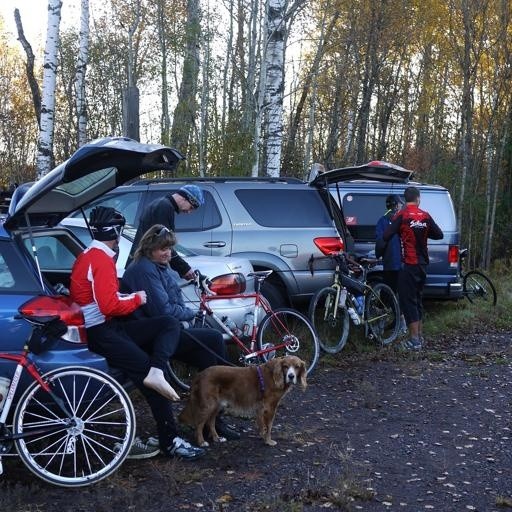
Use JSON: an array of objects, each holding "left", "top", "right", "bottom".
[
  {"left": 370, "top": 326, "right": 423, "bottom": 351},
  {"left": 112, "top": 436, "right": 207, "bottom": 462}
]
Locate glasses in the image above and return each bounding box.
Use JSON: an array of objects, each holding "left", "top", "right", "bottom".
[{"left": 152, "top": 226, "right": 174, "bottom": 242}]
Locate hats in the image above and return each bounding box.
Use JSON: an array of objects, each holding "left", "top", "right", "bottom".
[
  {"left": 175, "top": 184, "right": 205, "bottom": 211},
  {"left": 384, "top": 193, "right": 405, "bottom": 208}
]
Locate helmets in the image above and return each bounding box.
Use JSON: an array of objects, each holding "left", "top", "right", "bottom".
[{"left": 88, "top": 205, "right": 127, "bottom": 242}]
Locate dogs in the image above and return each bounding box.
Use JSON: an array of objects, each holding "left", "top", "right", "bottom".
[{"left": 176, "top": 354, "right": 309, "bottom": 447}]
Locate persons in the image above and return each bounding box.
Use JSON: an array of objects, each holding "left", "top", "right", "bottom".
[
  {"left": 375, "top": 193, "right": 409, "bottom": 337},
  {"left": 121, "top": 223, "right": 225, "bottom": 374},
  {"left": 70, "top": 206, "right": 205, "bottom": 461},
  {"left": 125, "top": 185, "right": 201, "bottom": 281},
  {"left": 383, "top": 187, "right": 443, "bottom": 351}
]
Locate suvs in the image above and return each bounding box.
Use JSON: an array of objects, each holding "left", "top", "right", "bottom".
[
  {"left": 1, "top": 135, "right": 186, "bottom": 403},
  {"left": 65, "top": 161, "right": 413, "bottom": 337}
]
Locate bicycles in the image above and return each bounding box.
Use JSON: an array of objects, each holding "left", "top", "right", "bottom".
[
  {"left": 304, "top": 248, "right": 400, "bottom": 355},
  {"left": 458, "top": 247, "right": 498, "bottom": 307},
  {"left": 189, "top": 267, "right": 319, "bottom": 381},
  {"left": 1, "top": 314, "right": 136, "bottom": 487}
]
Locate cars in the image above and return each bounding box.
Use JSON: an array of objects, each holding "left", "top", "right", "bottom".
[{"left": 58, "top": 218, "right": 262, "bottom": 342}]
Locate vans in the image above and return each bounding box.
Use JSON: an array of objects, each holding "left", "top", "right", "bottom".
[{"left": 326, "top": 182, "right": 463, "bottom": 303}]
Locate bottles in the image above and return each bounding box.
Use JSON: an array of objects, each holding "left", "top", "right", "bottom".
[
  {"left": 0, "top": 375, "right": 12, "bottom": 414},
  {"left": 339, "top": 286, "right": 347, "bottom": 306},
  {"left": 356, "top": 295, "right": 363, "bottom": 315},
  {"left": 221, "top": 316, "right": 243, "bottom": 338},
  {"left": 241, "top": 311, "right": 254, "bottom": 337},
  {"left": 347, "top": 292, "right": 359, "bottom": 308}
]
